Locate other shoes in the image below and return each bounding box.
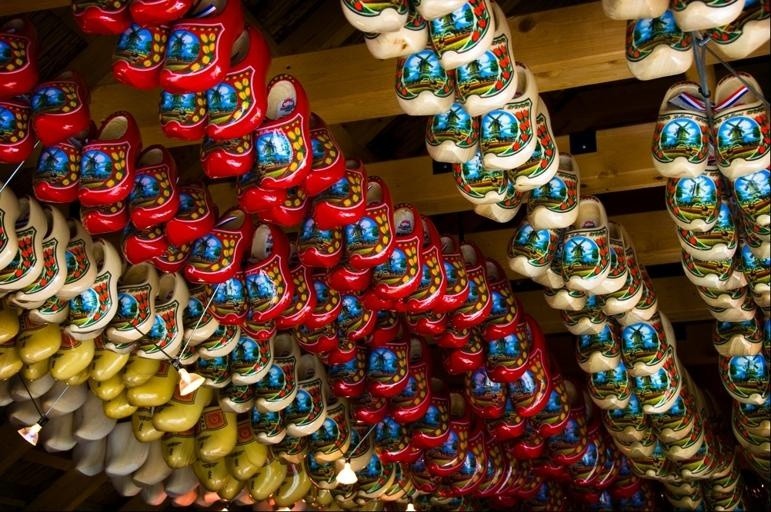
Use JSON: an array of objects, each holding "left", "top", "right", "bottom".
[{"left": 0, "top": 0, "right": 771, "bottom": 512}]
